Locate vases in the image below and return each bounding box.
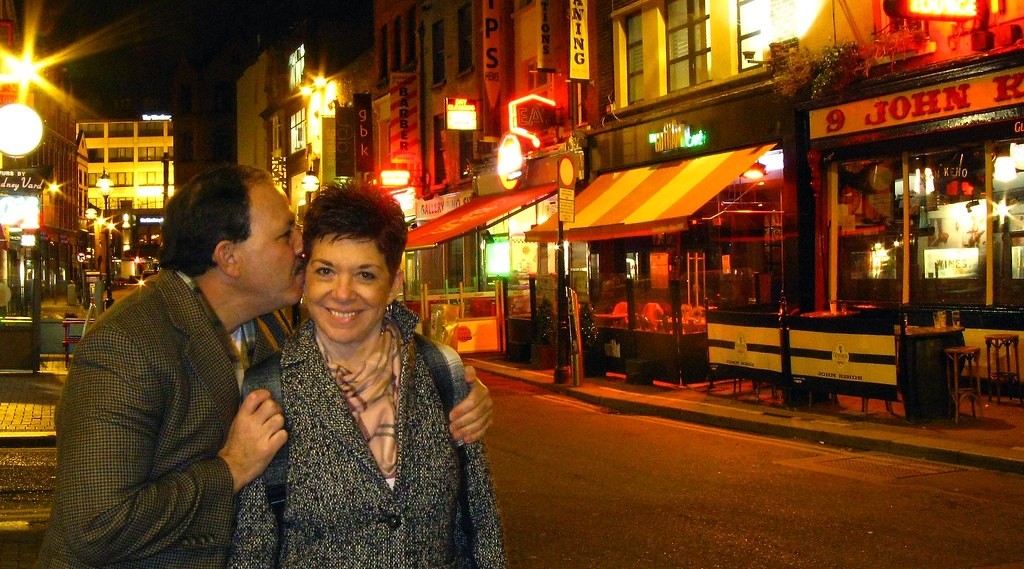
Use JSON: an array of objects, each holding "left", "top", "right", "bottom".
[{"left": 625, "top": 358, "right": 656, "bottom": 386}]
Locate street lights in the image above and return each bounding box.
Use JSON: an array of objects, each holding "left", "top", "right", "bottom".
[{"left": 96, "top": 166, "right": 115, "bottom": 311}]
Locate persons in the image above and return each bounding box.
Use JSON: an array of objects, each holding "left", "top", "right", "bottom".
[
  {"left": 38, "top": 169, "right": 491, "bottom": 569},
  {"left": 228, "top": 182, "right": 505, "bottom": 568}
]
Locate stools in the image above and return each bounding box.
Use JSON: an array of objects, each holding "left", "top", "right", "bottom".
[
  {"left": 944, "top": 346, "right": 984, "bottom": 424},
  {"left": 984, "top": 334, "right": 1023, "bottom": 404}
]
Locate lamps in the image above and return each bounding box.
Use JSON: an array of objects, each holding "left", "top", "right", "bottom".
[
  {"left": 743, "top": 51, "right": 770, "bottom": 64},
  {"left": 481, "top": 230, "right": 508, "bottom": 244}
]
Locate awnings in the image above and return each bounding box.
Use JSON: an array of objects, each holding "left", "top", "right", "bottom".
[
  {"left": 524, "top": 140, "right": 778, "bottom": 243},
  {"left": 403, "top": 183, "right": 558, "bottom": 249}
]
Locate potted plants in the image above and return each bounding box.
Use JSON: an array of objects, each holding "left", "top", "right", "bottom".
[{"left": 530, "top": 298, "right": 555, "bottom": 369}]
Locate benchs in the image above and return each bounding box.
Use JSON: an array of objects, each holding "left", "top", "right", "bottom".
[{"left": 62, "top": 320, "right": 95, "bottom": 368}]
